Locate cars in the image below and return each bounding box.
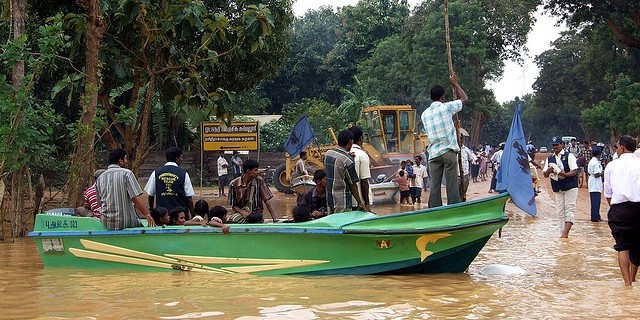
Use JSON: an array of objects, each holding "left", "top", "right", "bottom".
[{"left": 540, "top": 147, "right": 548, "bottom": 152}]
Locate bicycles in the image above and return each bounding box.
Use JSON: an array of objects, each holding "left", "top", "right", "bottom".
[{"left": 576, "top": 155, "right": 585, "bottom": 188}]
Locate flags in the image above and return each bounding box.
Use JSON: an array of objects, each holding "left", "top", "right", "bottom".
[
  {"left": 495, "top": 103, "right": 537, "bottom": 218},
  {"left": 284, "top": 116, "right": 321, "bottom": 160}
]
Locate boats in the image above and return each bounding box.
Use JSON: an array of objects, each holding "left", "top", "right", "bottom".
[
  {"left": 290, "top": 175, "right": 401, "bottom": 205},
  {"left": 29, "top": 192, "right": 511, "bottom": 275}
]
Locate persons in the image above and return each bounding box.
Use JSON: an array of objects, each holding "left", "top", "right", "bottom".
[
  {"left": 612, "top": 146, "right": 618, "bottom": 160},
  {"left": 182, "top": 199, "right": 210, "bottom": 227},
  {"left": 406, "top": 159, "right": 414, "bottom": 176},
  {"left": 551, "top": 142, "right": 568, "bottom": 157},
  {"left": 471, "top": 149, "right": 480, "bottom": 182},
  {"left": 151, "top": 207, "right": 170, "bottom": 227},
  {"left": 600, "top": 146, "right": 612, "bottom": 187},
  {"left": 576, "top": 141, "right": 591, "bottom": 187},
  {"left": 543, "top": 136, "right": 579, "bottom": 238},
  {"left": 477, "top": 150, "right": 481, "bottom": 159},
  {"left": 300, "top": 169, "right": 327, "bottom": 220},
  {"left": 604, "top": 135, "right": 640, "bottom": 288},
  {"left": 217, "top": 150, "right": 228, "bottom": 196},
  {"left": 393, "top": 170, "right": 412, "bottom": 205},
  {"left": 209, "top": 206, "right": 230, "bottom": 235},
  {"left": 528, "top": 155, "right": 542, "bottom": 193},
  {"left": 144, "top": 148, "right": 194, "bottom": 221},
  {"left": 421, "top": 73, "right": 470, "bottom": 208},
  {"left": 292, "top": 152, "right": 308, "bottom": 178},
  {"left": 324, "top": 129, "right": 369, "bottom": 216},
  {"left": 74, "top": 169, "right": 106, "bottom": 218},
  {"left": 169, "top": 207, "right": 187, "bottom": 225},
  {"left": 568, "top": 139, "right": 581, "bottom": 177},
  {"left": 481, "top": 152, "right": 487, "bottom": 181},
  {"left": 409, "top": 156, "right": 428, "bottom": 205},
  {"left": 292, "top": 205, "right": 310, "bottom": 222},
  {"left": 490, "top": 145, "right": 495, "bottom": 157},
  {"left": 231, "top": 150, "right": 243, "bottom": 180},
  {"left": 348, "top": 126, "right": 376, "bottom": 215},
  {"left": 227, "top": 159, "right": 279, "bottom": 224},
  {"left": 465, "top": 143, "right": 490, "bottom": 152},
  {"left": 527, "top": 141, "right": 535, "bottom": 152},
  {"left": 95, "top": 149, "right": 157, "bottom": 231},
  {"left": 396, "top": 161, "right": 408, "bottom": 179},
  {"left": 488, "top": 143, "right": 505, "bottom": 193},
  {"left": 587, "top": 146, "right": 604, "bottom": 222},
  {"left": 457, "top": 133, "right": 473, "bottom": 201}
]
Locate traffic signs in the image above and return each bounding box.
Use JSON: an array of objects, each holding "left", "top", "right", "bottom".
[{"left": 201, "top": 121, "right": 259, "bottom": 151}]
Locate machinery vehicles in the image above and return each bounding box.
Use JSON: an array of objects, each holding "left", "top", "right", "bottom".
[{"left": 273, "top": 106, "right": 430, "bottom": 193}]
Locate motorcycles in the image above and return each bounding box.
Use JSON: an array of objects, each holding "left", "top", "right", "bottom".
[
  {"left": 486, "top": 161, "right": 499, "bottom": 191},
  {"left": 530, "top": 160, "right": 545, "bottom": 197}
]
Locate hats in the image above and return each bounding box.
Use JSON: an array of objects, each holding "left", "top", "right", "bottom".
[
  {"left": 550, "top": 136, "right": 563, "bottom": 144},
  {"left": 93, "top": 169, "right": 106, "bottom": 178}
]
